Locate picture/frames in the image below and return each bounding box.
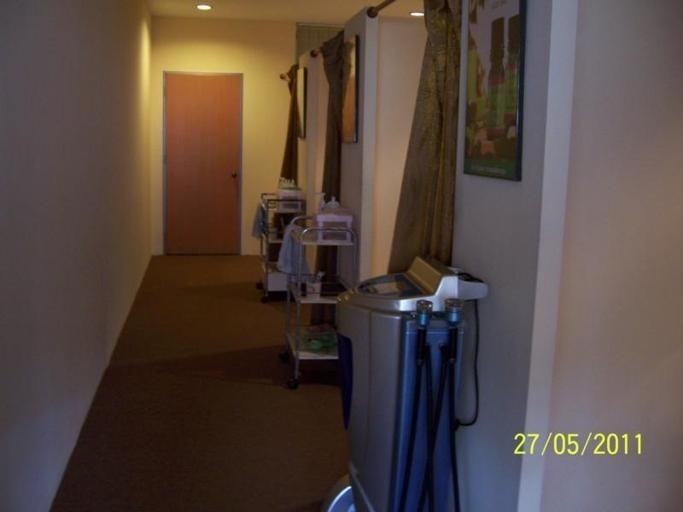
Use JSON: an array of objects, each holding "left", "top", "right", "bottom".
[
  {"left": 463, "top": 0, "right": 527, "bottom": 182},
  {"left": 338, "top": 31, "right": 359, "bottom": 143},
  {"left": 294, "top": 67, "right": 308, "bottom": 139}
]
